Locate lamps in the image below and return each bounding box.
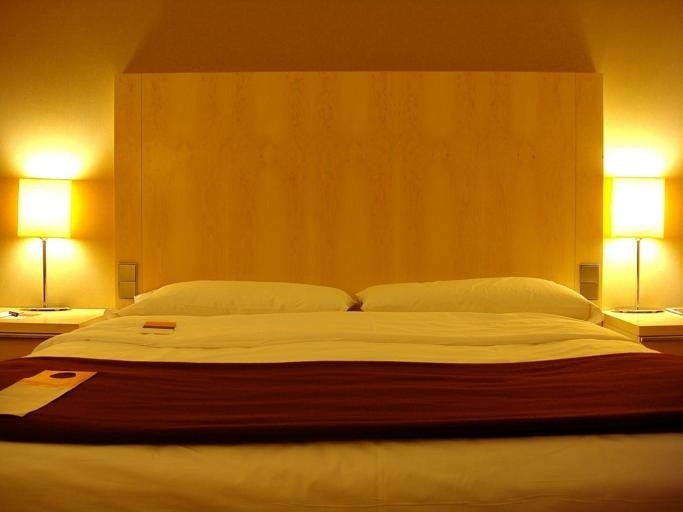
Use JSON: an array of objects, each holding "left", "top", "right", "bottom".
[
  {"left": 611, "top": 175, "right": 666, "bottom": 313},
  {"left": 16, "top": 178, "right": 72, "bottom": 312}
]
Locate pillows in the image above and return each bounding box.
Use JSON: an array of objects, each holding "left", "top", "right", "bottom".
[
  {"left": 112, "top": 279, "right": 360, "bottom": 328},
  {"left": 354, "top": 276, "right": 607, "bottom": 326}
]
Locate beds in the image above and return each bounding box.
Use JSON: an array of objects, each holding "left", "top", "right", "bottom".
[{"left": 0, "top": 70, "right": 682, "bottom": 512}]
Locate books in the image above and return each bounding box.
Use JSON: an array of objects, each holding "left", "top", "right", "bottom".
[{"left": 143, "top": 321, "right": 176, "bottom": 328}]
